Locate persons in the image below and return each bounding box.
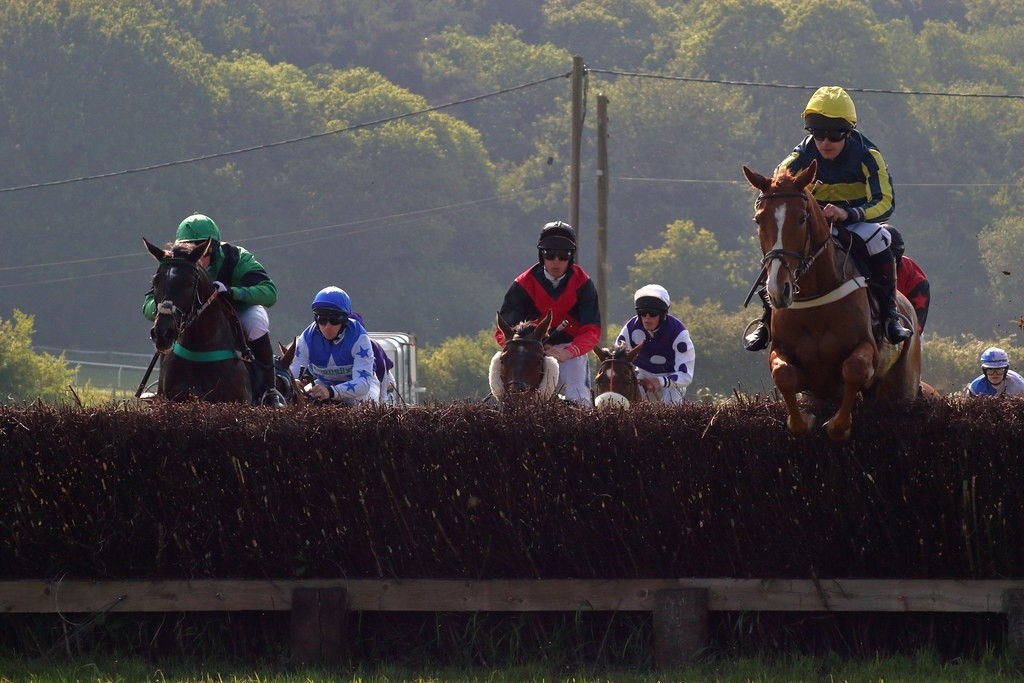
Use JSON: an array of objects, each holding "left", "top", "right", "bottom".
[
  {"left": 288, "top": 285, "right": 381, "bottom": 409},
  {"left": 743, "top": 86, "right": 915, "bottom": 351},
  {"left": 349, "top": 313, "right": 397, "bottom": 405},
  {"left": 143, "top": 212, "right": 277, "bottom": 410},
  {"left": 495, "top": 221, "right": 601, "bottom": 408},
  {"left": 965, "top": 347, "right": 1024, "bottom": 398},
  {"left": 614, "top": 284, "right": 696, "bottom": 408}
]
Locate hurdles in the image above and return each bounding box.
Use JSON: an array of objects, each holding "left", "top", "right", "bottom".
[{"left": 1, "top": 394, "right": 1024, "bottom": 668}]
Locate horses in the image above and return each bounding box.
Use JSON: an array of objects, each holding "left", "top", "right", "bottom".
[
  {"left": 489, "top": 308, "right": 561, "bottom": 411},
  {"left": 141, "top": 235, "right": 254, "bottom": 410},
  {"left": 263, "top": 336, "right": 315, "bottom": 411},
  {"left": 741, "top": 158, "right": 922, "bottom": 447},
  {"left": 592, "top": 339, "right": 648, "bottom": 413}
]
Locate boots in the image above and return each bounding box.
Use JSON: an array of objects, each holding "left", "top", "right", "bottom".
[
  {"left": 247, "top": 332, "right": 283, "bottom": 408},
  {"left": 872, "top": 258, "right": 913, "bottom": 345},
  {"left": 744, "top": 306, "right": 771, "bottom": 352}
]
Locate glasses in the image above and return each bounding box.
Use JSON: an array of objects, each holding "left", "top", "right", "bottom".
[
  {"left": 807, "top": 127, "right": 848, "bottom": 143},
  {"left": 986, "top": 367, "right": 1005, "bottom": 375},
  {"left": 541, "top": 249, "right": 572, "bottom": 261},
  {"left": 638, "top": 309, "right": 661, "bottom": 317},
  {"left": 313, "top": 314, "right": 344, "bottom": 326}
]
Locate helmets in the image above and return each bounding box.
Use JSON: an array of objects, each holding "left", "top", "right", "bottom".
[
  {"left": 634, "top": 284, "right": 671, "bottom": 323},
  {"left": 803, "top": 86, "right": 857, "bottom": 131},
  {"left": 981, "top": 347, "right": 1009, "bottom": 379},
  {"left": 881, "top": 223, "right": 905, "bottom": 258},
  {"left": 312, "top": 286, "right": 351, "bottom": 317},
  {"left": 537, "top": 220, "right": 576, "bottom": 265},
  {"left": 176, "top": 212, "right": 221, "bottom": 245}
]
